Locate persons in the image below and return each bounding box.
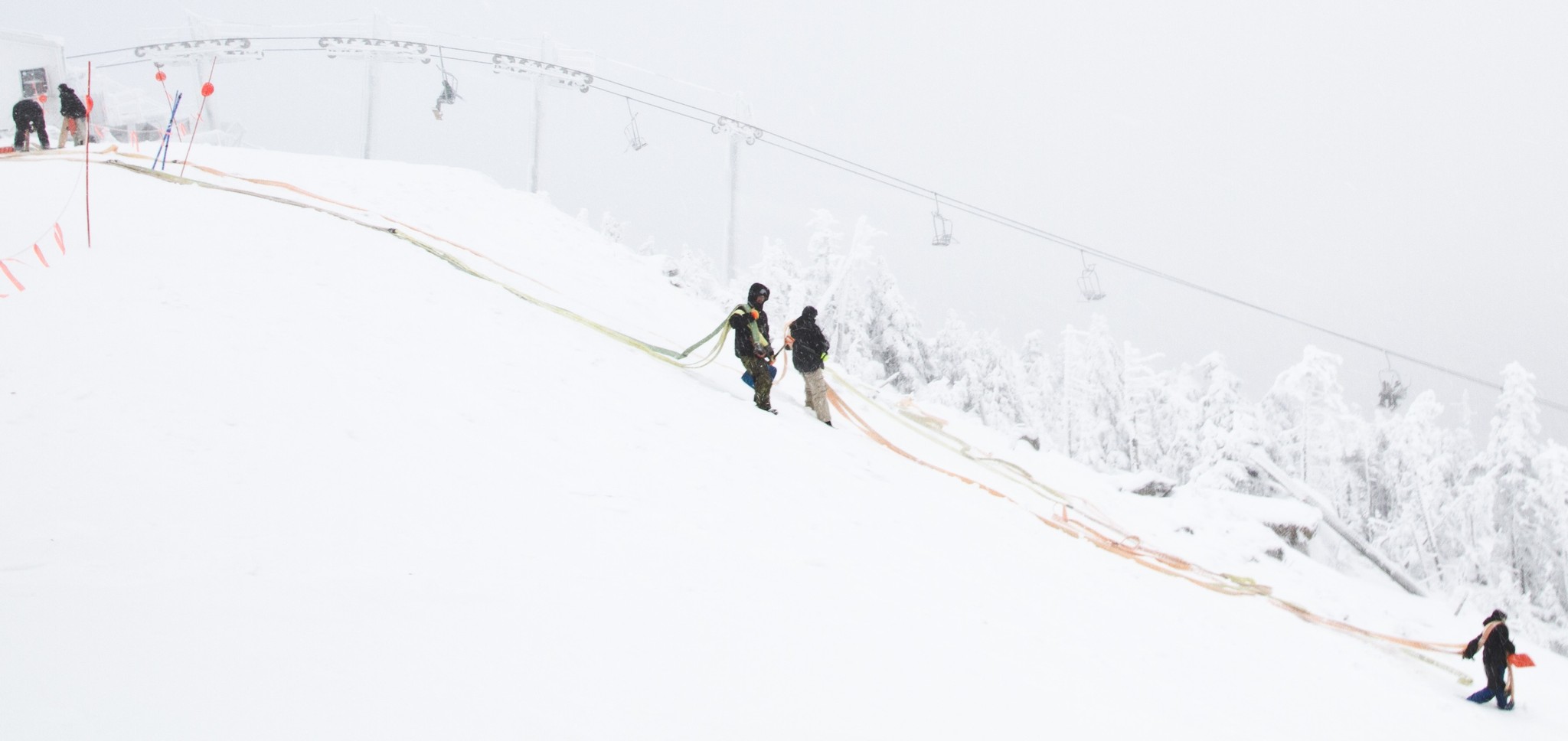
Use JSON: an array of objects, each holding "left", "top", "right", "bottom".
[
  {"left": 1463, "top": 610, "right": 1515, "bottom": 709},
  {"left": 58, "top": 83, "right": 86, "bottom": 148},
  {"left": 13, "top": 100, "right": 49, "bottom": 150},
  {"left": 785, "top": 307, "right": 830, "bottom": 425},
  {"left": 729, "top": 283, "right": 778, "bottom": 415}
]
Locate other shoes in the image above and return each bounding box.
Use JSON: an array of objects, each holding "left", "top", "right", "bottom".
[
  {"left": 826, "top": 420, "right": 832, "bottom": 427},
  {"left": 770, "top": 410, "right": 777, "bottom": 415}
]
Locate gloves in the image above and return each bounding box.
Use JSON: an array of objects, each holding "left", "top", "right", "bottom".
[
  {"left": 750, "top": 308, "right": 759, "bottom": 321},
  {"left": 768, "top": 353, "right": 776, "bottom": 365}
]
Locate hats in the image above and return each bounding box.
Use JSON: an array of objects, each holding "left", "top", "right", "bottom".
[{"left": 759, "top": 288, "right": 768, "bottom": 296}]
